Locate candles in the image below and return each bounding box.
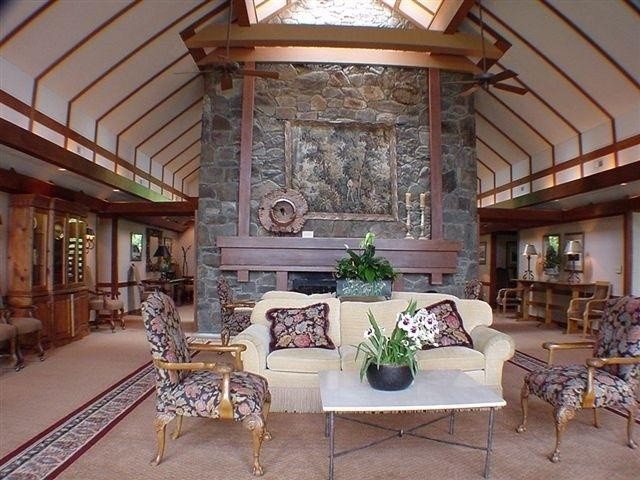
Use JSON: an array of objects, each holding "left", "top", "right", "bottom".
[
  {"left": 419, "top": 191, "right": 426, "bottom": 210},
  {"left": 404, "top": 190, "right": 413, "bottom": 210}
]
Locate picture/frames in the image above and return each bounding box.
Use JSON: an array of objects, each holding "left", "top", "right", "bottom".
[
  {"left": 164, "top": 237, "right": 173, "bottom": 248},
  {"left": 146, "top": 226, "right": 163, "bottom": 273},
  {"left": 506, "top": 241, "right": 517, "bottom": 270},
  {"left": 479, "top": 241, "right": 487, "bottom": 266},
  {"left": 130, "top": 231, "right": 144, "bottom": 262},
  {"left": 283, "top": 120, "right": 401, "bottom": 223},
  {"left": 562, "top": 231, "right": 586, "bottom": 272},
  {"left": 541, "top": 232, "right": 561, "bottom": 266}
]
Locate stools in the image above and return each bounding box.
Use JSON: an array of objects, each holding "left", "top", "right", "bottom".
[{"left": 0, "top": 309, "right": 22, "bottom": 373}]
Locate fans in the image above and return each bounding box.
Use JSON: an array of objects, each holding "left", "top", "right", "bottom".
[
  {"left": 171, "top": 0, "right": 282, "bottom": 96},
  {"left": 442, "top": 0, "right": 531, "bottom": 101}
]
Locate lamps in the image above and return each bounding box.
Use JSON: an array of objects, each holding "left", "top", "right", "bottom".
[
  {"left": 564, "top": 241, "right": 585, "bottom": 284},
  {"left": 85, "top": 228, "right": 96, "bottom": 253},
  {"left": 152, "top": 245, "right": 173, "bottom": 283},
  {"left": 520, "top": 243, "right": 539, "bottom": 281}
]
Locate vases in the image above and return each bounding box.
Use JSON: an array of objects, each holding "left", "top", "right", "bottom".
[{"left": 363, "top": 354, "right": 416, "bottom": 392}]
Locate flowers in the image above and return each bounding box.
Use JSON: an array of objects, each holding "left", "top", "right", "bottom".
[{"left": 354, "top": 297, "right": 441, "bottom": 385}]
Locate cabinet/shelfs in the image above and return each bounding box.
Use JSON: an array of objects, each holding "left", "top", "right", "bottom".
[
  {"left": 50, "top": 198, "right": 93, "bottom": 349},
  {"left": 1, "top": 192, "right": 56, "bottom": 352}
]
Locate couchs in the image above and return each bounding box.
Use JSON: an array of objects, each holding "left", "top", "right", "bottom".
[{"left": 221, "top": 284, "right": 517, "bottom": 418}]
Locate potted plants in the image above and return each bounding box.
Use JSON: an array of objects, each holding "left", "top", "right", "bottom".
[
  {"left": 332, "top": 231, "right": 398, "bottom": 304},
  {"left": 544, "top": 246, "right": 563, "bottom": 275}
]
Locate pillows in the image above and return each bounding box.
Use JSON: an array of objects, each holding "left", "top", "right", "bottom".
[
  {"left": 398, "top": 297, "right": 475, "bottom": 353},
  {"left": 265, "top": 302, "right": 336, "bottom": 353}
]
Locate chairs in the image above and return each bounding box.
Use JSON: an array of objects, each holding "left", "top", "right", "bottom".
[
  {"left": 515, "top": 294, "right": 640, "bottom": 464},
  {"left": 136, "top": 288, "right": 273, "bottom": 476},
  {"left": 496, "top": 275, "right": 527, "bottom": 320},
  {"left": 0, "top": 294, "right": 47, "bottom": 373},
  {"left": 566, "top": 279, "right": 612, "bottom": 335},
  {"left": 462, "top": 277, "right": 485, "bottom": 302},
  {"left": 213, "top": 275, "right": 262, "bottom": 355},
  {"left": 84, "top": 264, "right": 128, "bottom": 333}
]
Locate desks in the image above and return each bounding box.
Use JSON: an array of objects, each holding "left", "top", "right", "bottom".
[{"left": 510, "top": 276, "right": 613, "bottom": 331}]
